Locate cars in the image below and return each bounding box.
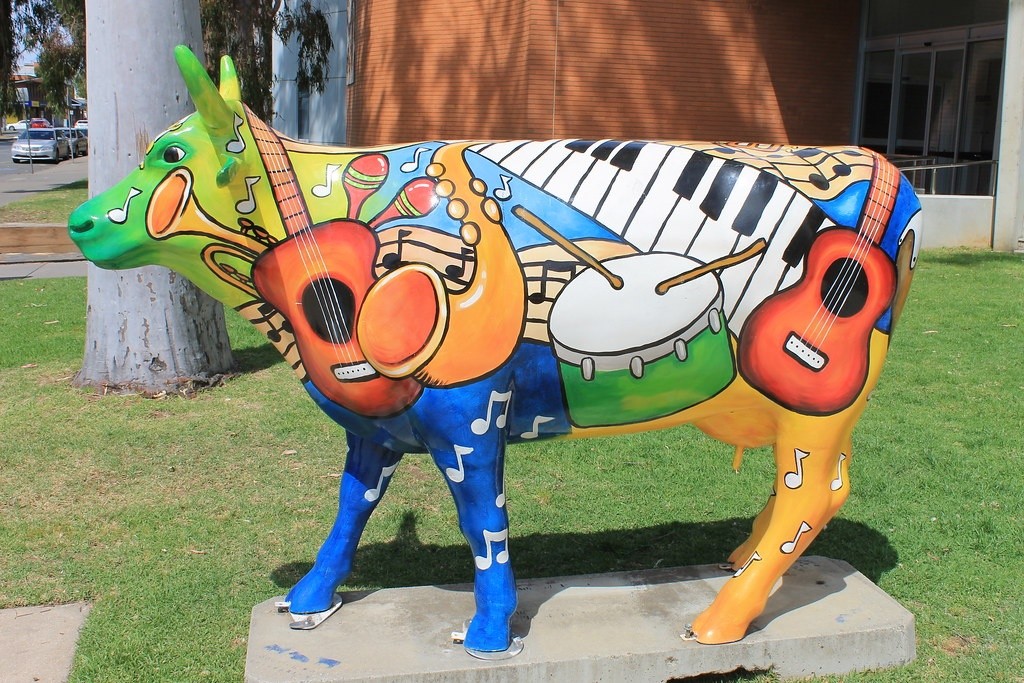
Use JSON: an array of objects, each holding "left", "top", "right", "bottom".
[{"left": 7, "top": 117, "right": 88, "bottom": 163}]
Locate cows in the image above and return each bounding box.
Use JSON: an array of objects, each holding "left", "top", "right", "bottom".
[{"left": 67, "top": 44, "right": 922, "bottom": 664}]
